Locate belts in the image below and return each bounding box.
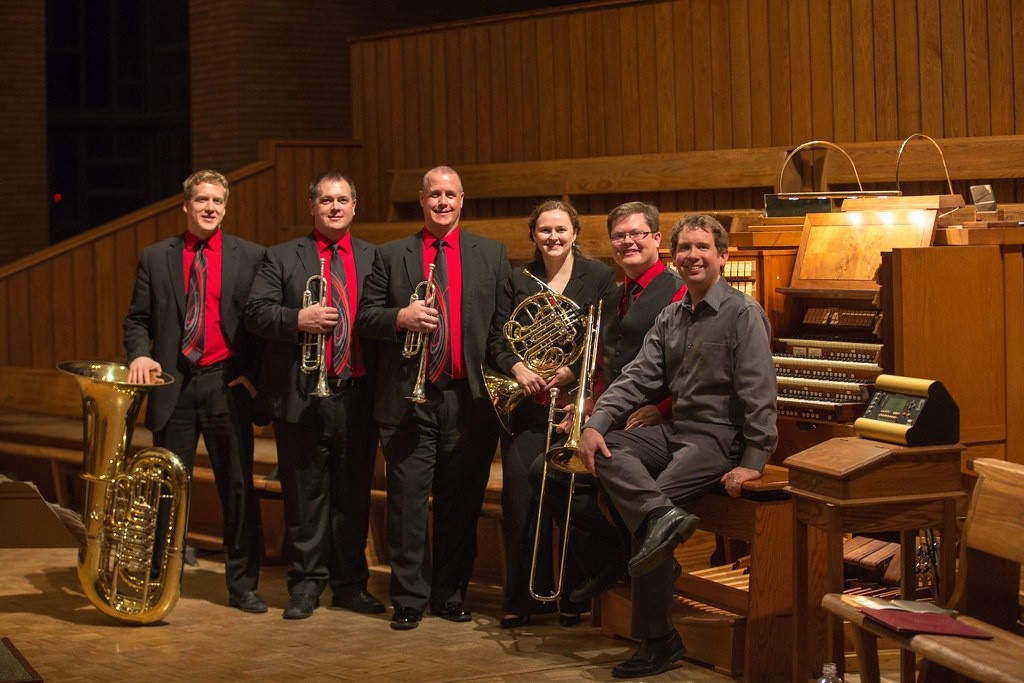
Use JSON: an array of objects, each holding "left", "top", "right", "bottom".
[
  {"left": 327, "top": 377, "right": 369, "bottom": 390},
  {"left": 421, "top": 377, "right": 470, "bottom": 391}
]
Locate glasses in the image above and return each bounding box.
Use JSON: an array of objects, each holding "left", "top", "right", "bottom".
[{"left": 610, "top": 229, "right": 657, "bottom": 241}]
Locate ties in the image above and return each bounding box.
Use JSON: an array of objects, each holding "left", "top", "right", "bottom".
[
  {"left": 180, "top": 240, "right": 208, "bottom": 365},
  {"left": 323, "top": 241, "right": 357, "bottom": 382},
  {"left": 425, "top": 239, "right": 454, "bottom": 392},
  {"left": 618, "top": 280, "right": 640, "bottom": 321}
]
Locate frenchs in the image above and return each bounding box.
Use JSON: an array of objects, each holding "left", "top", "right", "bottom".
[{"left": 480, "top": 268, "right": 588, "bottom": 436}]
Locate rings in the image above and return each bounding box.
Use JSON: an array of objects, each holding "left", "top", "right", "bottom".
[{"left": 732, "top": 480, "right": 736, "bottom": 482}]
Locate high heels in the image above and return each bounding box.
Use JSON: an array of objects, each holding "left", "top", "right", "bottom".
[
  {"left": 499, "top": 612, "right": 531, "bottom": 629},
  {"left": 559, "top": 613, "right": 582, "bottom": 627}
]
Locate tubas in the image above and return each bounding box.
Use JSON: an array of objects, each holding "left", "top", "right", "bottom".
[{"left": 54, "top": 360, "right": 191, "bottom": 624}]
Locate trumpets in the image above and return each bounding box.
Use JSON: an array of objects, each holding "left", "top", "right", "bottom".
[
  {"left": 401, "top": 264, "right": 436, "bottom": 405},
  {"left": 298, "top": 258, "right": 337, "bottom": 398}
]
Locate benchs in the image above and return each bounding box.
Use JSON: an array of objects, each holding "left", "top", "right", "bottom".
[{"left": 601, "top": 465, "right": 826, "bottom": 683}]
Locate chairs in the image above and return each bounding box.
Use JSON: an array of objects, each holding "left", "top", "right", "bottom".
[{"left": 821, "top": 458, "right": 1024, "bottom": 683}]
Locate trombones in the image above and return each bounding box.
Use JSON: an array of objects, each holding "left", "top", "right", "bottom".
[{"left": 528, "top": 298, "right": 602, "bottom": 604}]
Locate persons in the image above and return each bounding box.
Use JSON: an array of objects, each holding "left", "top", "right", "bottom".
[
  {"left": 556, "top": 201, "right": 688, "bottom": 606},
  {"left": 487, "top": 201, "right": 618, "bottom": 628},
  {"left": 353, "top": 165, "right": 512, "bottom": 627},
  {"left": 123, "top": 171, "right": 269, "bottom": 612},
  {"left": 244, "top": 172, "right": 387, "bottom": 617},
  {"left": 577, "top": 212, "right": 779, "bottom": 678}
]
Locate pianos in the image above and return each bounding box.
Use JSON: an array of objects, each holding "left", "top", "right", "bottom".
[{"left": 770, "top": 286, "right": 887, "bottom": 423}]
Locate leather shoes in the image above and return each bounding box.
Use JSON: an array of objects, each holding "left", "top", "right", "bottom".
[
  {"left": 229, "top": 590, "right": 268, "bottom": 613},
  {"left": 282, "top": 593, "right": 320, "bottom": 619},
  {"left": 673, "top": 557, "right": 684, "bottom": 583},
  {"left": 628, "top": 506, "right": 702, "bottom": 578},
  {"left": 390, "top": 606, "right": 423, "bottom": 629},
  {"left": 611, "top": 627, "right": 686, "bottom": 679},
  {"left": 431, "top": 606, "right": 472, "bottom": 622},
  {"left": 569, "top": 564, "right": 619, "bottom": 604},
  {"left": 332, "top": 588, "right": 387, "bottom": 614}
]
